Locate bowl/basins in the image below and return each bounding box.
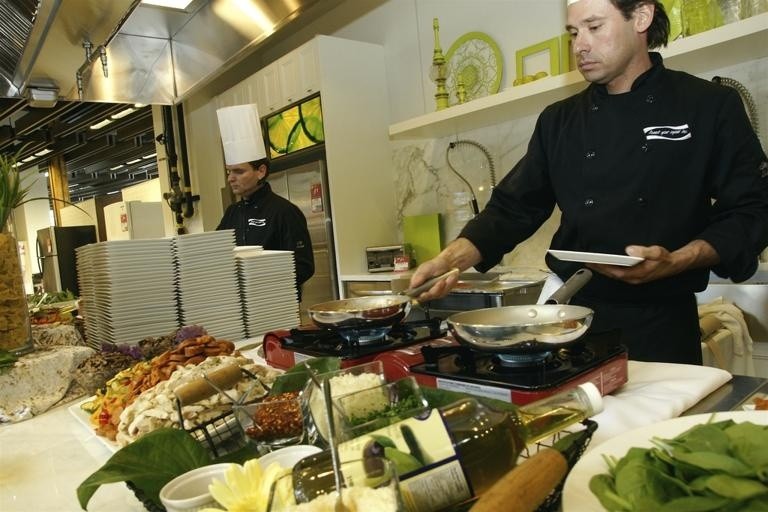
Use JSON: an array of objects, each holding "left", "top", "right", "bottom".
[{"left": 158, "top": 445, "right": 322, "bottom": 511}]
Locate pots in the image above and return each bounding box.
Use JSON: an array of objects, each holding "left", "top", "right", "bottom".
[{"left": 307, "top": 268, "right": 595, "bottom": 353}]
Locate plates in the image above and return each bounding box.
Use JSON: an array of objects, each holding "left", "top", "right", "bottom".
[
  {"left": 560, "top": 411, "right": 767, "bottom": 511},
  {"left": 75, "top": 228, "right": 300, "bottom": 351},
  {"left": 546, "top": 248, "right": 647, "bottom": 267}
]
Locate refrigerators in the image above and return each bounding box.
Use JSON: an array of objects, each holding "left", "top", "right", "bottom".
[
  {"left": 36, "top": 226, "right": 96, "bottom": 293},
  {"left": 102, "top": 200, "right": 163, "bottom": 240}
]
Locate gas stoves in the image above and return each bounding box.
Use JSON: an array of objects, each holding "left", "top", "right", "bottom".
[{"left": 265, "top": 318, "right": 628, "bottom": 405}]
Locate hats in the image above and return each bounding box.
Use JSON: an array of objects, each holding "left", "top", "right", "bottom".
[{"left": 215, "top": 102, "right": 269, "bottom": 166}]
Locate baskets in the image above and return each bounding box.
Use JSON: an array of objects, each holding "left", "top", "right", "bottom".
[{"left": 128, "top": 364, "right": 597, "bottom": 512}]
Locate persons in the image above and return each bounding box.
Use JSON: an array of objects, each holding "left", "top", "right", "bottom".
[
  {"left": 408, "top": 0, "right": 768, "bottom": 366},
  {"left": 215, "top": 158, "right": 315, "bottom": 303}
]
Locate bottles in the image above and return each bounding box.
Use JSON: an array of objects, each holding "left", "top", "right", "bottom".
[{"left": 292, "top": 381, "right": 606, "bottom": 511}]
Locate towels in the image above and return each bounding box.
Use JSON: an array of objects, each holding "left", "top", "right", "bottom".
[
  {"left": 696, "top": 296, "right": 754, "bottom": 356},
  {"left": 553, "top": 360, "right": 732, "bottom": 447}
]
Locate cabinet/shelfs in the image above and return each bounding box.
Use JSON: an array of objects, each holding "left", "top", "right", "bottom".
[{"left": 343, "top": 279, "right": 410, "bottom": 298}]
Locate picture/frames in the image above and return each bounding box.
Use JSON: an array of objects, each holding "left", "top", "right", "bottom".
[
  {"left": 515, "top": 36, "right": 560, "bottom": 83},
  {"left": 560, "top": 32, "right": 576, "bottom": 74}
]
